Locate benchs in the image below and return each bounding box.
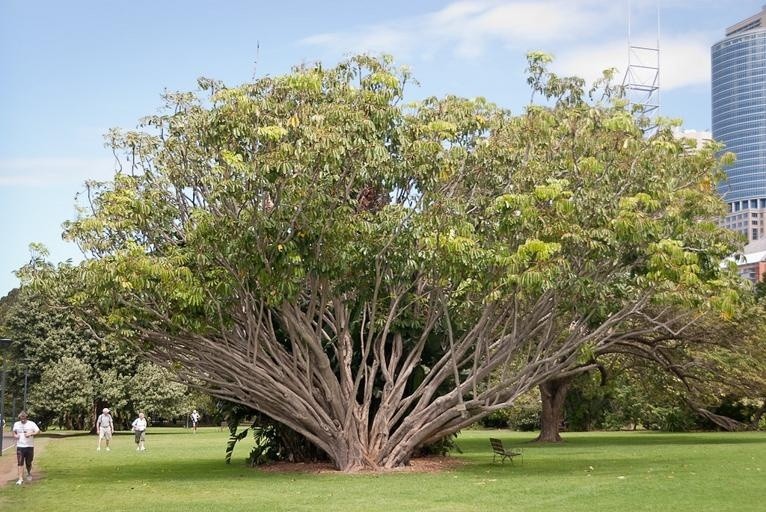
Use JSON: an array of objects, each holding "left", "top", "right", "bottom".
[{"left": 490, "top": 438, "right": 521, "bottom": 465}]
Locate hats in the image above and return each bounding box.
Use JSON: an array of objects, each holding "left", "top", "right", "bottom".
[{"left": 103, "top": 408, "right": 111, "bottom": 413}]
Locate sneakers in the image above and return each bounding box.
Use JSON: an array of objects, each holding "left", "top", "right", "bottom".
[
  {"left": 97, "top": 446, "right": 146, "bottom": 452},
  {"left": 16, "top": 472, "right": 32, "bottom": 486}
]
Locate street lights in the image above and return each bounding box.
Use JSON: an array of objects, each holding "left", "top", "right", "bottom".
[
  {"left": 0, "top": 338, "right": 13, "bottom": 457},
  {"left": 21, "top": 359, "right": 34, "bottom": 412}
]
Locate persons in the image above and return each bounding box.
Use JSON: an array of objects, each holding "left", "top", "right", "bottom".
[
  {"left": 132, "top": 413, "right": 147, "bottom": 450},
  {"left": 190, "top": 410, "right": 201, "bottom": 431},
  {"left": 58, "top": 411, "right": 95, "bottom": 431},
  {"left": 97, "top": 408, "right": 114, "bottom": 451},
  {"left": 13, "top": 411, "right": 40, "bottom": 485}
]
